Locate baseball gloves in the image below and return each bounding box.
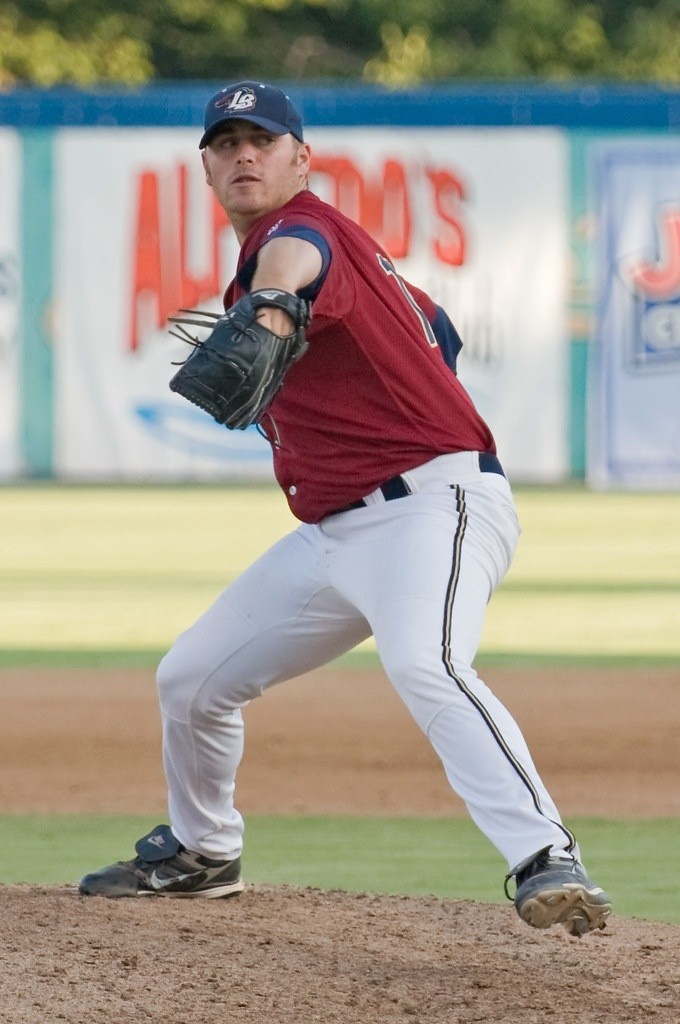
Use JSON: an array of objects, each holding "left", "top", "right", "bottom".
[{"left": 168, "top": 288, "right": 314, "bottom": 431}]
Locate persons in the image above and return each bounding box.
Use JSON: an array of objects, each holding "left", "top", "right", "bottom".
[{"left": 78, "top": 81, "right": 612, "bottom": 937}]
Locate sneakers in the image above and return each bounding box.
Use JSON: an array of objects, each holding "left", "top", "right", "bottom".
[
  {"left": 503, "top": 845, "right": 612, "bottom": 936},
  {"left": 79, "top": 824, "right": 244, "bottom": 900}
]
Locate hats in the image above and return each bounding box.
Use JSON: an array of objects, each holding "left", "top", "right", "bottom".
[{"left": 198, "top": 81, "right": 305, "bottom": 150}]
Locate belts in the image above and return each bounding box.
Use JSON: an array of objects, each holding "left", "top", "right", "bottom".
[{"left": 325, "top": 454, "right": 505, "bottom": 516}]
